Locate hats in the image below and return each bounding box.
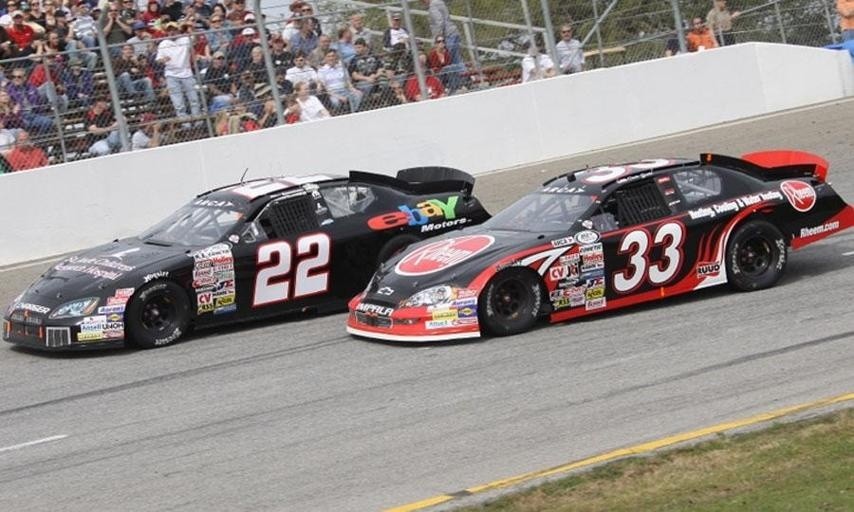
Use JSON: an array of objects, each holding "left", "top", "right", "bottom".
[
  {"left": 242, "top": 28, "right": 256, "bottom": 35},
  {"left": 269, "top": 33, "right": 287, "bottom": 48},
  {"left": 165, "top": 22, "right": 178, "bottom": 30},
  {"left": 254, "top": 82, "right": 272, "bottom": 98},
  {"left": 133, "top": 22, "right": 147, "bottom": 29},
  {"left": 245, "top": 14, "right": 255, "bottom": 22},
  {"left": 12, "top": 10, "right": 25, "bottom": 19},
  {"left": 54, "top": 10, "right": 69, "bottom": 17},
  {"left": 214, "top": 51, "right": 225, "bottom": 59},
  {"left": 180, "top": 21, "right": 195, "bottom": 30},
  {"left": 289, "top": 0, "right": 305, "bottom": 10},
  {"left": 392, "top": 12, "right": 403, "bottom": 20}
]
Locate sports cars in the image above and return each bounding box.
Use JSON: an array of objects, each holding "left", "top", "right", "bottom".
[
  {"left": 346, "top": 144, "right": 854, "bottom": 350},
  {"left": 2, "top": 164, "right": 497, "bottom": 358}
]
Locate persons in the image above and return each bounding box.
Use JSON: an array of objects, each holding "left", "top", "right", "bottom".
[
  {"left": 835, "top": 0, "right": 854, "bottom": 41},
  {"left": 521, "top": 46, "right": 556, "bottom": 82},
  {"left": 1, "top": 0, "right": 469, "bottom": 172},
  {"left": 663, "top": 38, "right": 681, "bottom": 56},
  {"left": 705, "top": 0, "right": 740, "bottom": 46},
  {"left": 236, "top": 210, "right": 259, "bottom": 239},
  {"left": 685, "top": 16, "right": 715, "bottom": 52},
  {"left": 555, "top": 23, "right": 586, "bottom": 74}
]
